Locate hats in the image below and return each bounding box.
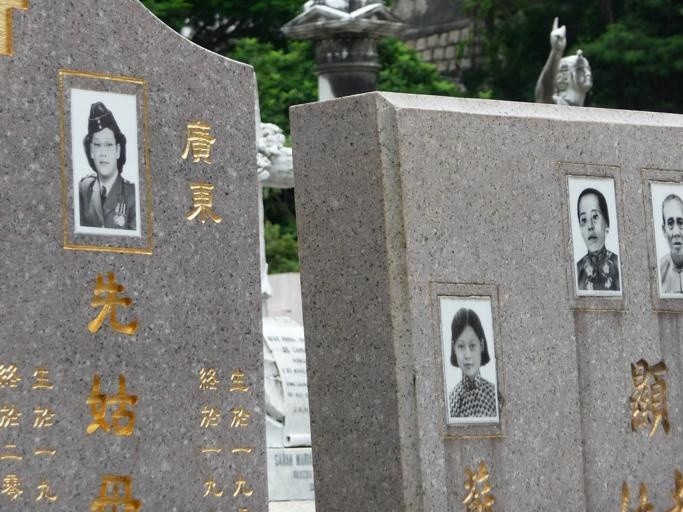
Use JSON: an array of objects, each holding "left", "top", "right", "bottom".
[{"left": 87, "top": 101, "right": 114, "bottom": 135}]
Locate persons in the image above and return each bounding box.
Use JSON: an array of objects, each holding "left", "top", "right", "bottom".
[
  {"left": 658, "top": 194, "right": 683, "bottom": 293},
  {"left": 449, "top": 307, "right": 496, "bottom": 418},
  {"left": 576, "top": 188, "right": 620, "bottom": 291},
  {"left": 535, "top": 16, "right": 593, "bottom": 107},
  {"left": 79, "top": 101, "right": 136, "bottom": 231}
]
